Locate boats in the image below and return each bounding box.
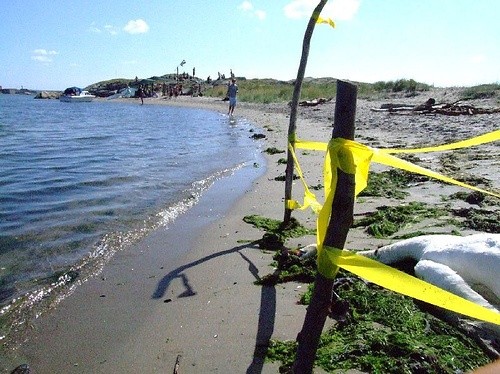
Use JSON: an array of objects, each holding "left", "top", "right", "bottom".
[{"left": 59, "top": 91, "right": 96, "bottom": 102}]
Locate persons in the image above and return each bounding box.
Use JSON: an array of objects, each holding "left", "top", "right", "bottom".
[
  {"left": 134, "top": 66, "right": 236, "bottom": 105},
  {"left": 225, "top": 79, "right": 239, "bottom": 115}
]
